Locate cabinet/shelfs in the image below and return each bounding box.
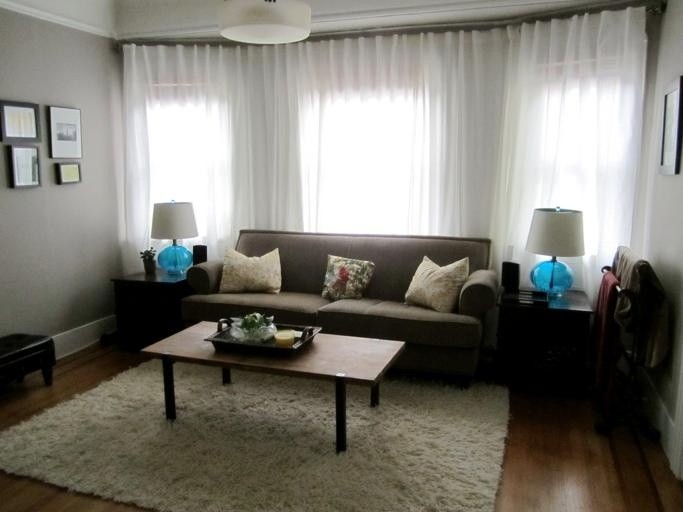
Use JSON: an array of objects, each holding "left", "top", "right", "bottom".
[
  {"left": 110, "top": 269, "right": 188, "bottom": 348},
  {"left": 500, "top": 288, "right": 598, "bottom": 402}
]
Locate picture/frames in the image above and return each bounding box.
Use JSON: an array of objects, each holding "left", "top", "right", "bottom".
[
  {"left": 0, "top": 99, "right": 42, "bottom": 146},
  {"left": 658, "top": 74, "right": 682, "bottom": 178},
  {"left": 54, "top": 161, "right": 82, "bottom": 186},
  {"left": 7, "top": 144, "right": 41, "bottom": 190},
  {"left": 45, "top": 105, "right": 82, "bottom": 159}
]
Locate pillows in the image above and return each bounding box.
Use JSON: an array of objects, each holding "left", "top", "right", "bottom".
[
  {"left": 323, "top": 253, "right": 376, "bottom": 302},
  {"left": 215, "top": 246, "right": 282, "bottom": 295},
  {"left": 405, "top": 253, "right": 470, "bottom": 314}
]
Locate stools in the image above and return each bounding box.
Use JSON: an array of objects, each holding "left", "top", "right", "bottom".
[{"left": 1, "top": 332, "right": 56, "bottom": 393}]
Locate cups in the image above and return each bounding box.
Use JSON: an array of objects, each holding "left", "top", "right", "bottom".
[
  {"left": 274, "top": 332, "right": 291, "bottom": 347},
  {"left": 279, "top": 329, "right": 295, "bottom": 345}
]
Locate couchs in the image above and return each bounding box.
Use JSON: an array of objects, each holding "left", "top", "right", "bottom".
[{"left": 180, "top": 228, "right": 500, "bottom": 392}]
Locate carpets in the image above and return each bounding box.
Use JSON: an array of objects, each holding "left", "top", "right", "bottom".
[{"left": 0, "top": 357, "right": 513, "bottom": 511}]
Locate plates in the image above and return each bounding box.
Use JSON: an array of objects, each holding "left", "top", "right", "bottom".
[{"left": 203, "top": 315, "right": 323, "bottom": 359}]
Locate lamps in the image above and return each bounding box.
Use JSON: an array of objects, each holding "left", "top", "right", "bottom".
[
  {"left": 523, "top": 205, "right": 588, "bottom": 294},
  {"left": 149, "top": 199, "right": 200, "bottom": 278},
  {"left": 214, "top": 1, "right": 316, "bottom": 47}
]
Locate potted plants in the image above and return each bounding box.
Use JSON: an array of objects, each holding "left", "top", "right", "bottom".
[{"left": 139, "top": 245, "right": 157, "bottom": 274}]
